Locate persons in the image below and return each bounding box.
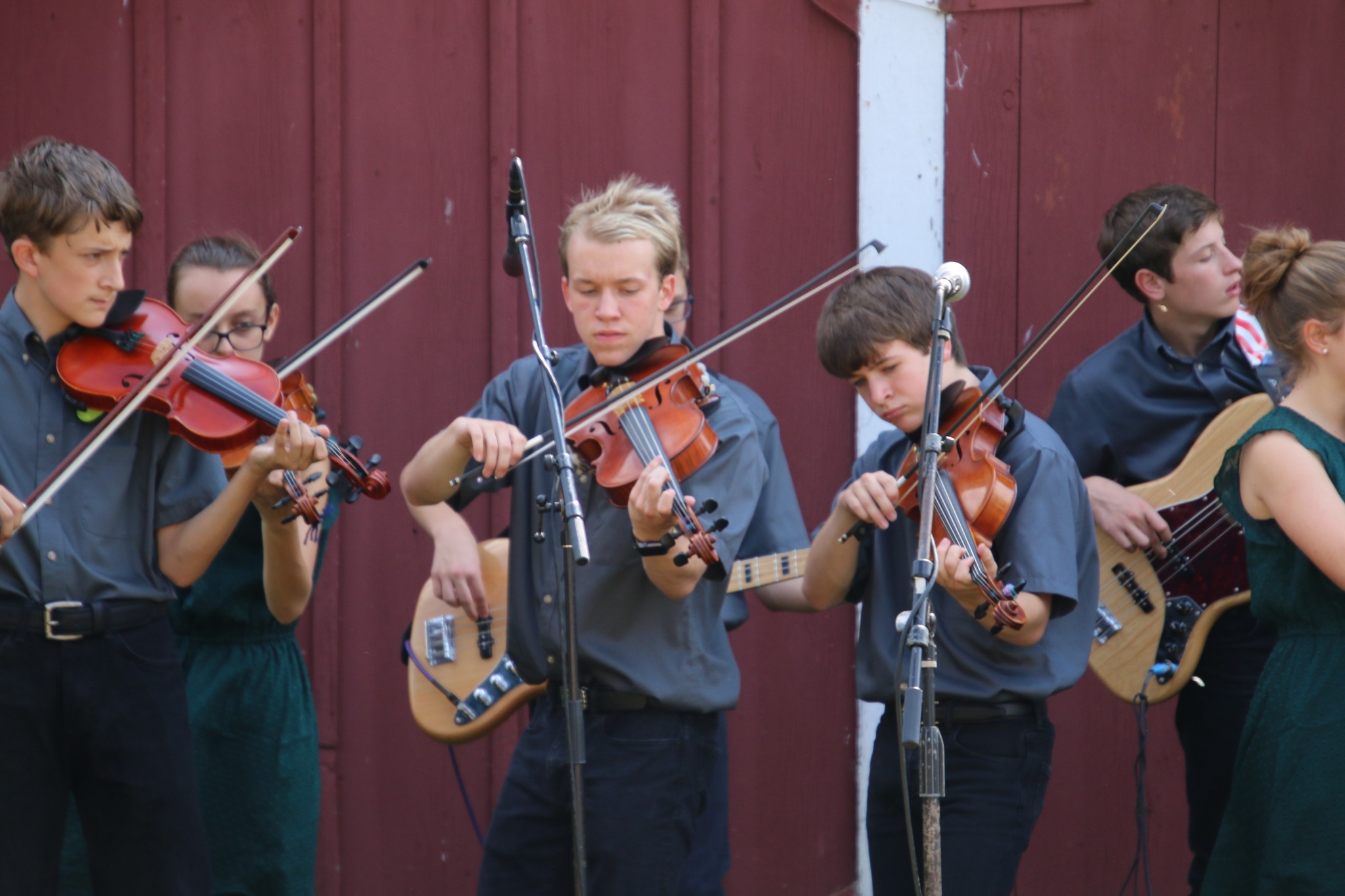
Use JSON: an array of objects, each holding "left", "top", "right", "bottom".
[
  {"left": 1046, "top": 184, "right": 1301, "bottom": 896},
  {"left": 0, "top": 134, "right": 330, "bottom": 896},
  {"left": 398, "top": 174, "right": 821, "bottom": 896},
  {"left": 155, "top": 234, "right": 345, "bottom": 896},
  {"left": 801, "top": 264, "right": 1100, "bottom": 896},
  {"left": 1197, "top": 227, "right": 1345, "bottom": 896}
]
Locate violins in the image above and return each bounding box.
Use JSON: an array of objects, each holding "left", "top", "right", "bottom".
[
  {"left": 54, "top": 295, "right": 395, "bottom": 505},
  {"left": 216, "top": 354, "right": 332, "bottom": 529},
  {"left": 894, "top": 385, "right": 1029, "bottom": 638},
  {"left": 561, "top": 342, "right": 730, "bottom": 567}
]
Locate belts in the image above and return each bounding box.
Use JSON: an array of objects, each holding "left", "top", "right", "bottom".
[
  {"left": 529, "top": 681, "right": 664, "bottom": 715},
  {"left": 883, "top": 694, "right": 1048, "bottom": 724},
  {"left": 0, "top": 599, "right": 175, "bottom": 640}
]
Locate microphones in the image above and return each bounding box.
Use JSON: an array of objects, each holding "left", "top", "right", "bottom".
[
  {"left": 932, "top": 261, "right": 971, "bottom": 303},
  {"left": 501, "top": 157, "right": 525, "bottom": 278}
]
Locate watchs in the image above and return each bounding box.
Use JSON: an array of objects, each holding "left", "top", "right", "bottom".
[{"left": 628, "top": 530, "right": 669, "bottom": 556}]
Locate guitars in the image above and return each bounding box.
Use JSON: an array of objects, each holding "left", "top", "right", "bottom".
[
  {"left": 1084, "top": 389, "right": 1280, "bottom": 707},
  {"left": 405, "top": 532, "right": 817, "bottom": 748}
]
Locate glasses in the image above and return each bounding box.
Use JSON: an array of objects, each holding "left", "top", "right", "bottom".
[
  {"left": 178, "top": 311, "right": 269, "bottom": 356},
  {"left": 654, "top": 267, "right": 695, "bottom": 326}
]
